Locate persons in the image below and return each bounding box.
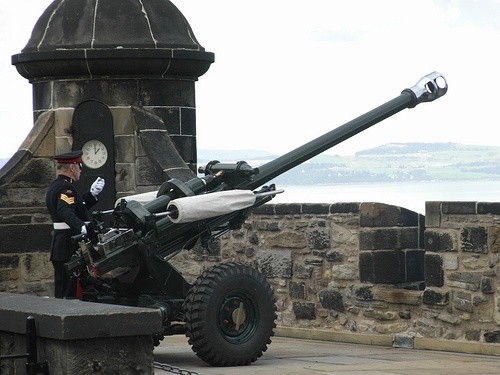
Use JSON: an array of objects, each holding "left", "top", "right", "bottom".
[{"left": 44, "top": 150, "right": 107, "bottom": 300}]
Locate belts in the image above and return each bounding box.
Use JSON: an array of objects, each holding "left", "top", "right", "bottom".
[{"left": 53, "top": 222, "right": 88, "bottom": 229}]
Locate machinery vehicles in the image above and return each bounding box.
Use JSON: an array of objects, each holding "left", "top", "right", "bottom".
[{"left": 64, "top": 69, "right": 450, "bottom": 368}]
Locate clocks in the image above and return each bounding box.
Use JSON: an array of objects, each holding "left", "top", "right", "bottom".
[{"left": 81, "top": 139, "right": 109, "bottom": 169}]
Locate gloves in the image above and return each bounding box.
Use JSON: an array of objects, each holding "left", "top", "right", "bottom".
[
  {"left": 81, "top": 225, "right": 87, "bottom": 234},
  {"left": 89, "top": 176, "right": 105, "bottom": 197}
]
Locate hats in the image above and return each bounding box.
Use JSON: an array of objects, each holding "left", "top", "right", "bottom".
[{"left": 54, "top": 150, "right": 83, "bottom": 164}]
